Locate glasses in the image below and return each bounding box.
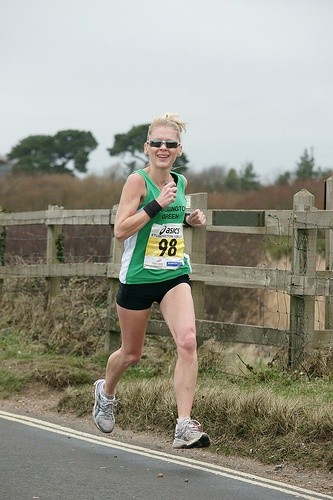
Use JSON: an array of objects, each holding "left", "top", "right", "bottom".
[{"left": 146, "top": 138, "right": 182, "bottom": 150}]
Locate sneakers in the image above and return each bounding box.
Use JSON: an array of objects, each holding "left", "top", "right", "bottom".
[
  {"left": 92, "top": 378, "right": 118, "bottom": 437},
  {"left": 172, "top": 417, "right": 212, "bottom": 450}
]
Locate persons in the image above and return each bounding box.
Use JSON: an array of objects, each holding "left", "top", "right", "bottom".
[{"left": 92, "top": 113, "right": 212, "bottom": 449}]
